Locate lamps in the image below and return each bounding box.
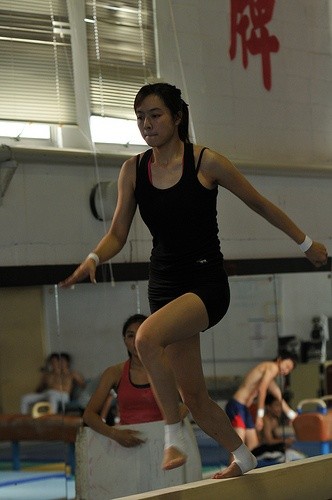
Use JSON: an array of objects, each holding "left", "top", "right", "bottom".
[{"left": 89, "top": 181, "right": 118, "bottom": 222}]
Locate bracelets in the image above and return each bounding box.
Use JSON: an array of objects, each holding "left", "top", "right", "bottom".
[
  {"left": 256, "top": 408, "right": 264, "bottom": 416},
  {"left": 297, "top": 234, "right": 312, "bottom": 252},
  {"left": 87, "top": 252, "right": 99, "bottom": 266},
  {"left": 286, "top": 408, "right": 294, "bottom": 418}
]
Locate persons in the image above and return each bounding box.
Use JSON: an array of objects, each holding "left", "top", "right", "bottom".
[
  {"left": 59, "top": 82, "right": 328, "bottom": 479},
  {"left": 82, "top": 314, "right": 188, "bottom": 447},
  {"left": 20, "top": 351, "right": 86, "bottom": 414},
  {"left": 224, "top": 355, "right": 298, "bottom": 451}
]
